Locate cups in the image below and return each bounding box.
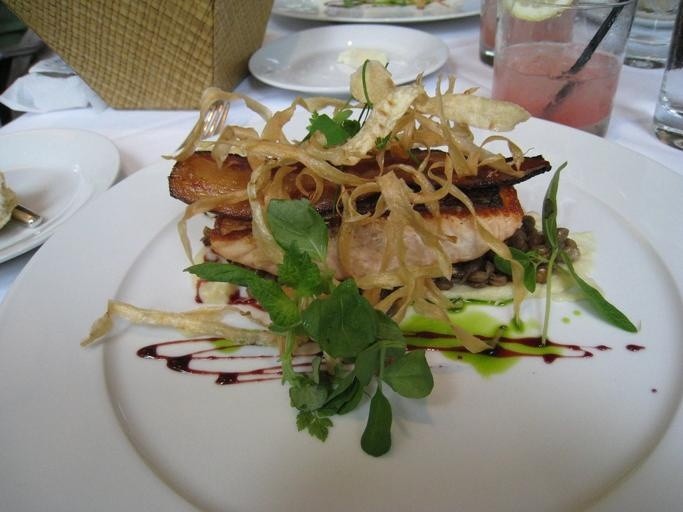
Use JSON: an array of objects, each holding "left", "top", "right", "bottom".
[{"left": 0, "top": 105, "right": 683, "bottom": 511}]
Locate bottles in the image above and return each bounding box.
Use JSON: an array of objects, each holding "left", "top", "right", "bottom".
[{"left": 476, "top": 1, "right": 683, "bottom": 150}]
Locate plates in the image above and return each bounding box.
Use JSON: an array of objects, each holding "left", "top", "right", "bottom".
[
  {"left": 265, "top": 0, "right": 495, "bottom": 25},
  {"left": 0, "top": 118, "right": 118, "bottom": 267},
  {"left": 248, "top": 21, "right": 451, "bottom": 94}
]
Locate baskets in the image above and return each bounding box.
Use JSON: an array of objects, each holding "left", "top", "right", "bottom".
[{"left": 3, "top": 0, "right": 273, "bottom": 110}]
